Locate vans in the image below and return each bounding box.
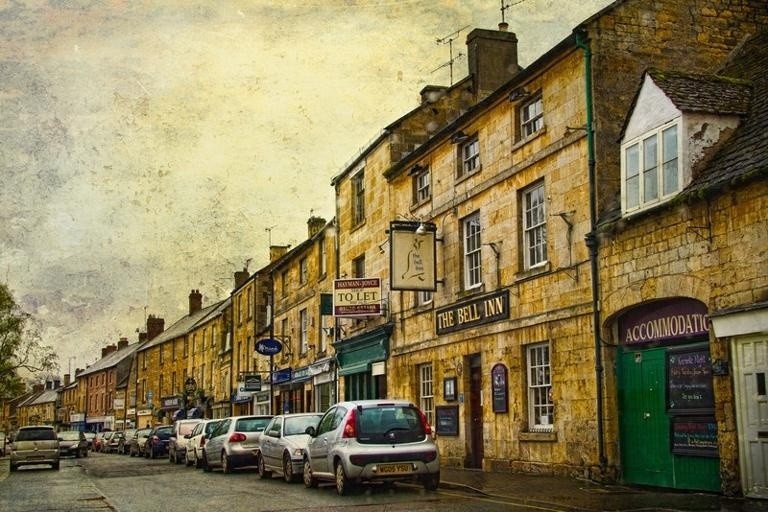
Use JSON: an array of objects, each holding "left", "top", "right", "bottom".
[{"left": 8, "top": 424, "right": 62, "bottom": 475}]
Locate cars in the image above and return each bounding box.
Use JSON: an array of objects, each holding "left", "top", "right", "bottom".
[
  {"left": 256, "top": 412, "right": 324, "bottom": 483},
  {"left": 300, "top": 398, "right": 441, "bottom": 498},
  {"left": 79, "top": 416, "right": 230, "bottom": 471},
  {"left": 0, "top": 431, "right": 8, "bottom": 459},
  {"left": 201, "top": 415, "right": 276, "bottom": 474},
  {"left": 56, "top": 429, "right": 88, "bottom": 460}
]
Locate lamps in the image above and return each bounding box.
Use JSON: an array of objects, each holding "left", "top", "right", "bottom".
[{"left": 411, "top": 215, "right": 426, "bottom": 235}]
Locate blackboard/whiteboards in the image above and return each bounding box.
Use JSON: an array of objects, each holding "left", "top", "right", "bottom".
[
  {"left": 491, "top": 363, "right": 508, "bottom": 413},
  {"left": 664, "top": 344, "right": 719, "bottom": 458},
  {"left": 435, "top": 405, "right": 459, "bottom": 436}
]
